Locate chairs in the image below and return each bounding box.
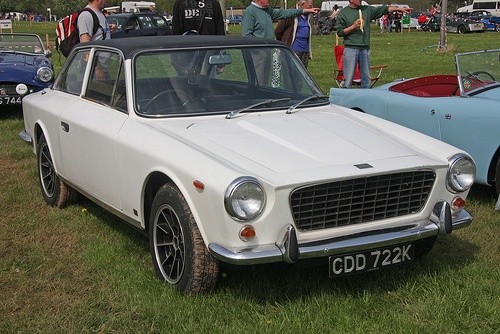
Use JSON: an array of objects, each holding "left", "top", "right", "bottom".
[{"left": 332, "top": 45, "right": 388, "bottom": 88}]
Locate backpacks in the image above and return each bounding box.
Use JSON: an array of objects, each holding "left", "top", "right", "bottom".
[{"left": 55, "top": 8, "right": 101, "bottom": 57}]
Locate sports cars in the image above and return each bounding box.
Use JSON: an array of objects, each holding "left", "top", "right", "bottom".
[{"left": 329, "top": 49, "right": 500, "bottom": 200}]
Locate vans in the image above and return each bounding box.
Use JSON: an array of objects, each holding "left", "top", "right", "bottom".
[
  {"left": 457, "top": 0, "right": 500, "bottom": 19},
  {"left": 321, "top": 0, "right": 370, "bottom": 13}
]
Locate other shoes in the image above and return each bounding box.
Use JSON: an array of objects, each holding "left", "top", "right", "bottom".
[{"left": 329, "top": 30, "right": 332, "bottom": 32}]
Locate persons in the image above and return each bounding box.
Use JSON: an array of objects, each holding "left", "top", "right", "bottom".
[
  {"left": 163, "top": 11, "right": 168, "bottom": 20},
  {"left": 149, "top": 5, "right": 158, "bottom": 13},
  {"left": 76, "top": 0, "right": 117, "bottom": 81},
  {"left": 170, "top": 0, "right": 225, "bottom": 77},
  {"left": 241, "top": 0, "right": 441, "bottom": 94},
  {"left": 103, "top": 9, "right": 109, "bottom": 16}
]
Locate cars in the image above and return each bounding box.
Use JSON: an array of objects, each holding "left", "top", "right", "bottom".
[
  {"left": 21, "top": 35, "right": 476, "bottom": 296},
  {"left": 0, "top": 32, "right": 56, "bottom": 107},
  {"left": 401, "top": 9, "right": 500, "bottom": 34}
]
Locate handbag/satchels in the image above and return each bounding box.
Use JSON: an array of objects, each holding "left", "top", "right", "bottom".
[{"left": 171, "top": 30, "right": 206, "bottom": 76}]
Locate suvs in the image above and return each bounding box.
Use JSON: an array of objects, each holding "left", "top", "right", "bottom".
[{"left": 103, "top": 11, "right": 171, "bottom": 39}]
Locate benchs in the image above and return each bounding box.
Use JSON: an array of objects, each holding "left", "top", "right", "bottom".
[{"left": 74, "top": 74, "right": 206, "bottom": 99}]
